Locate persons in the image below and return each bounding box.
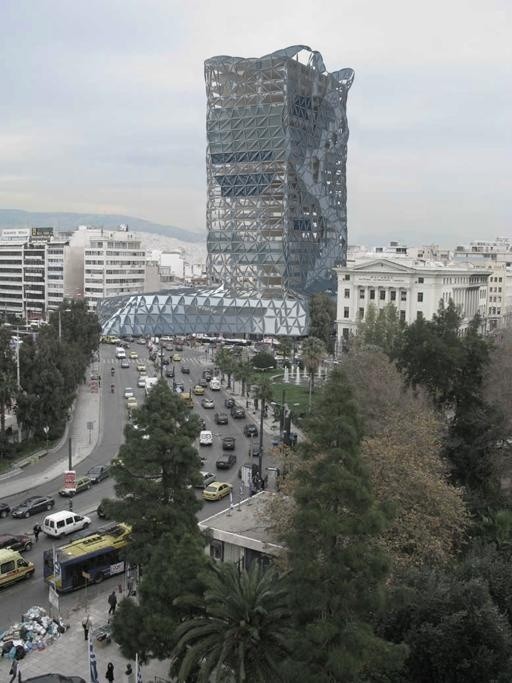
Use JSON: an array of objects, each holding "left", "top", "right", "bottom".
[
  {"left": 81, "top": 614, "right": 93, "bottom": 640},
  {"left": 8, "top": 656, "right": 22, "bottom": 683},
  {"left": 105, "top": 662, "right": 115, "bottom": 683},
  {"left": 6, "top": 398, "right": 18, "bottom": 415},
  {"left": 124, "top": 664, "right": 135, "bottom": 683},
  {"left": 111, "top": 384, "right": 114, "bottom": 394},
  {"left": 214, "top": 366, "right": 224, "bottom": 384},
  {"left": 126, "top": 576, "right": 137, "bottom": 597},
  {"left": 33, "top": 523, "right": 43, "bottom": 543},
  {"left": 68, "top": 496, "right": 73, "bottom": 512},
  {"left": 111, "top": 366, "right": 115, "bottom": 376},
  {"left": 270, "top": 437, "right": 290, "bottom": 462},
  {"left": 107, "top": 592, "right": 117, "bottom": 615},
  {"left": 117, "top": 583, "right": 122, "bottom": 593},
  {"left": 7, "top": 424, "right": 19, "bottom": 444}
]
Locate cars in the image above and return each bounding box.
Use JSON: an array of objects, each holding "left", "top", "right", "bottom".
[
  {"left": 100, "top": 334, "right": 260, "bottom": 501},
  {"left": 22, "top": 673, "right": 86, "bottom": 683},
  {"left": 0, "top": 464, "right": 132, "bottom": 595}
]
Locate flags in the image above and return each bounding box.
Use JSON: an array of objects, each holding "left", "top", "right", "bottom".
[
  {"left": 137, "top": 665, "right": 144, "bottom": 683},
  {"left": 88, "top": 638, "right": 99, "bottom": 683}
]
